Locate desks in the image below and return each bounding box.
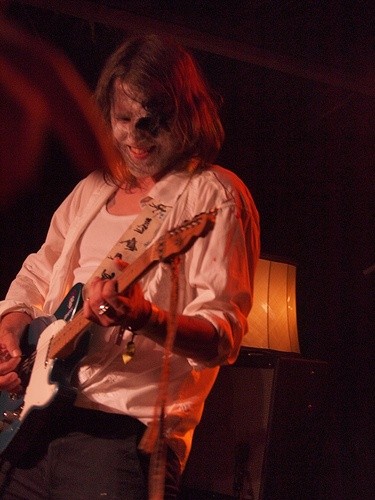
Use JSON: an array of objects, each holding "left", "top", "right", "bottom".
[{"left": 180, "top": 358, "right": 330, "bottom": 500}]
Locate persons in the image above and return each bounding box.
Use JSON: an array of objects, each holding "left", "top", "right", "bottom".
[{"left": 1, "top": 33, "right": 263, "bottom": 500}]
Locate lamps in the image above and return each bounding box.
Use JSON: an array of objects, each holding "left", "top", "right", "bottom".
[{"left": 239, "top": 258, "right": 302, "bottom": 357}]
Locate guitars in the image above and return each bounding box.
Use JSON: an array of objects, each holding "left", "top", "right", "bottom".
[{"left": 0, "top": 206, "right": 223, "bottom": 462}]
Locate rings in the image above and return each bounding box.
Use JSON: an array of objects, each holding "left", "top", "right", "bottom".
[{"left": 96, "top": 304, "right": 110, "bottom": 316}]
